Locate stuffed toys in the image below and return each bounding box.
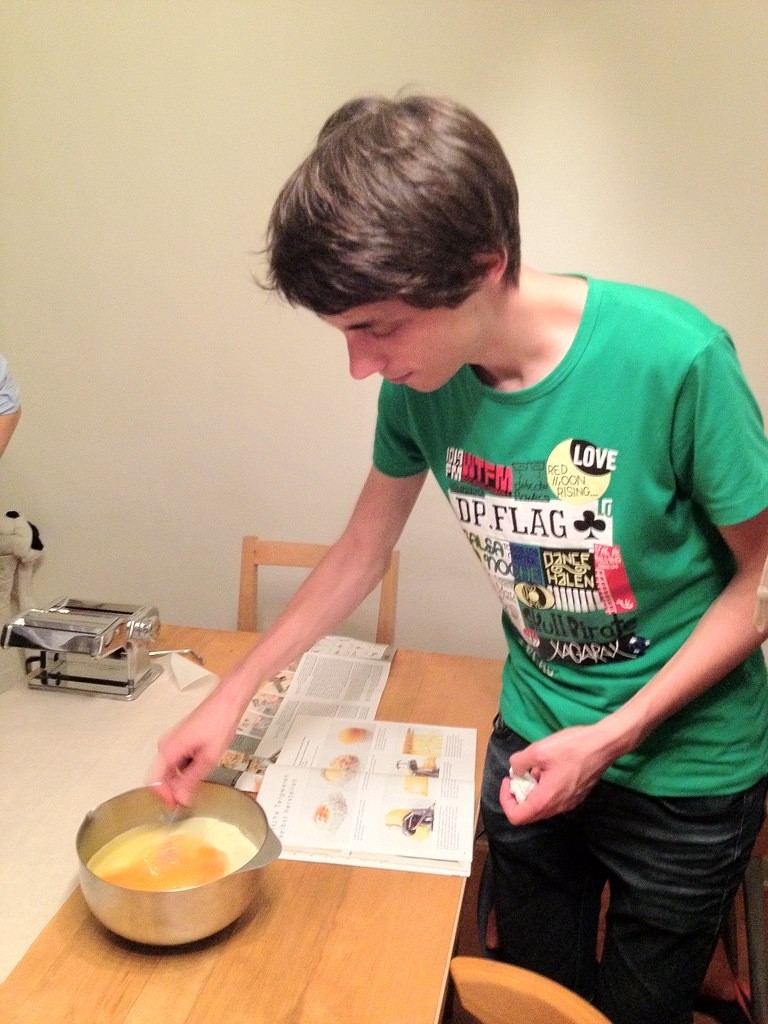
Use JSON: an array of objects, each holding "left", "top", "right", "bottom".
[{"left": 1, "top": 510, "right": 45, "bottom": 563}]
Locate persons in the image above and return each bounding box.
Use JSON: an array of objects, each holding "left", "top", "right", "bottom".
[
  {"left": 148, "top": 96, "right": 767, "bottom": 1024},
  {"left": 0, "top": 355, "right": 22, "bottom": 466}
]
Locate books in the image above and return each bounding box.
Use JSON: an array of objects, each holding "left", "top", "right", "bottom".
[{"left": 253, "top": 712, "right": 482, "bottom": 880}]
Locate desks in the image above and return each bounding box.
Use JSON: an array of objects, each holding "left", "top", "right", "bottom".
[{"left": 0, "top": 625, "right": 505, "bottom": 1024}]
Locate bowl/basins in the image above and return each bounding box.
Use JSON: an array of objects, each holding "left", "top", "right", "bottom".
[{"left": 73, "top": 778, "right": 283, "bottom": 947}]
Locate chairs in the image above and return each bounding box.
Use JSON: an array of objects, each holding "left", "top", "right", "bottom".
[
  {"left": 472, "top": 848, "right": 768, "bottom": 1024},
  {"left": 236, "top": 536, "right": 401, "bottom": 648}
]
